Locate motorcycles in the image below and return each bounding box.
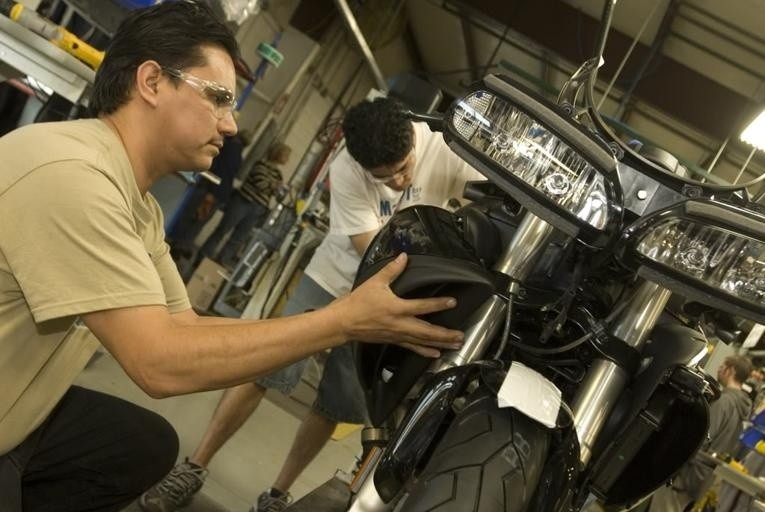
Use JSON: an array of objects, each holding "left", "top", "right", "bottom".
[{"left": 347, "top": 1, "right": 765, "bottom": 512}]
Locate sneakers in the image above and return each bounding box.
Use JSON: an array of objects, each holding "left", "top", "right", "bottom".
[
  {"left": 250, "top": 488, "right": 292, "bottom": 511},
  {"left": 141, "top": 465, "right": 209, "bottom": 510}
]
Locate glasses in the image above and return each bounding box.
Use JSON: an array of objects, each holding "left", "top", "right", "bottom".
[
  {"left": 136, "top": 57, "right": 239, "bottom": 119},
  {"left": 362, "top": 147, "right": 419, "bottom": 185}
]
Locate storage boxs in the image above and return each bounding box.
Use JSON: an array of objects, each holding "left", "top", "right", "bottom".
[{"left": 186, "top": 257, "right": 228, "bottom": 313}]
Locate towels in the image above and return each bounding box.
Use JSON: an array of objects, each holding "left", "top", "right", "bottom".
[{"left": 496, "top": 359, "right": 564, "bottom": 429}]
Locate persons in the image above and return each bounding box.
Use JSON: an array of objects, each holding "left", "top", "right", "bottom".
[
  {"left": 138, "top": 96, "right": 491, "bottom": 511},
  {"left": 746, "top": 367, "right": 765, "bottom": 414},
  {"left": 191, "top": 139, "right": 293, "bottom": 270},
  {"left": 0, "top": 0, "right": 465, "bottom": 510},
  {"left": 629, "top": 354, "right": 753, "bottom": 511},
  {"left": 172, "top": 129, "right": 255, "bottom": 237}
]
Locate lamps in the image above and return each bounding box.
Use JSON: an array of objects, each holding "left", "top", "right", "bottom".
[{"left": 737, "top": 103, "right": 765, "bottom": 155}]
